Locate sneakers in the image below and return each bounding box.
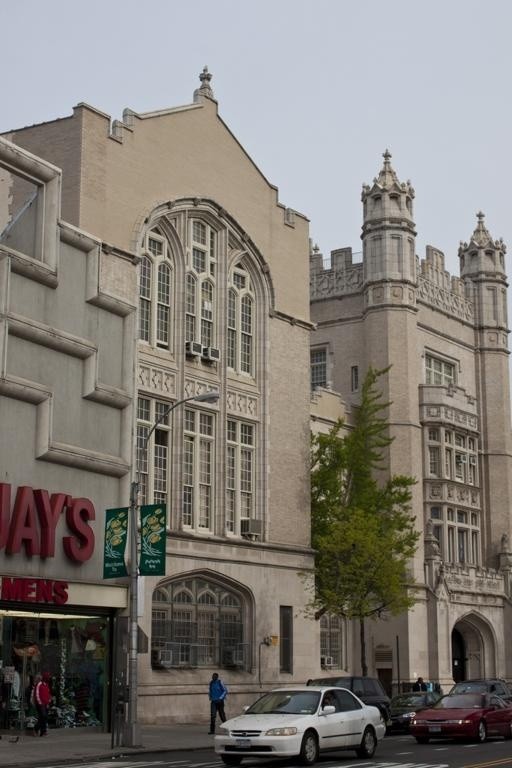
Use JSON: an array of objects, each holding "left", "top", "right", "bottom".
[
  {"left": 208, "top": 729, "right": 215, "bottom": 735},
  {"left": 32, "top": 726, "right": 49, "bottom": 737}
]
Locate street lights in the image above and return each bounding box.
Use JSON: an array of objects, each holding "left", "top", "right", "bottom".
[{"left": 121, "top": 392, "right": 222, "bottom": 748}]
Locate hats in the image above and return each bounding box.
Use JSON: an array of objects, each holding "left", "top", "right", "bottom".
[{"left": 42, "top": 672, "right": 50, "bottom": 679}]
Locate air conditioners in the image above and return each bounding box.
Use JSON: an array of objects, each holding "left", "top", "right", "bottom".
[
  {"left": 202, "top": 347, "right": 220, "bottom": 362},
  {"left": 153, "top": 647, "right": 175, "bottom": 667},
  {"left": 456, "top": 455, "right": 467, "bottom": 464},
  {"left": 469, "top": 457, "right": 476, "bottom": 466},
  {"left": 242, "top": 518, "right": 262, "bottom": 536},
  {"left": 322, "top": 656, "right": 333, "bottom": 667},
  {"left": 228, "top": 650, "right": 245, "bottom": 666},
  {"left": 185, "top": 340, "right": 203, "bottom": 357}
]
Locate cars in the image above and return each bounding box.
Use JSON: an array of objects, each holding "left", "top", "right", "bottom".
[
  {"left": 450, "top": 678, "right": 511, "bottom": 704},
  {"left": 409, "top": 692, "right": 512, "bottom": 744},
  {"left": 213, "top": 685, "right": 388, "bottom": 764},
  {"left": 308, "top": 676, "right": 393, "bottom": 728},
  {"left": 389, "top": 692, "right": 442, "bottom": 733}
]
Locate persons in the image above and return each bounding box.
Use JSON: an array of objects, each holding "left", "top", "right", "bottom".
[
  {"left": 412, "top": 676, "right": 428, "bottom": 692},
  {"left": 208, "top": 673, "right": 227, "bottom": 735},
  {"left": 31, "top": 670, "right": 55, "bottom": 739}
]
